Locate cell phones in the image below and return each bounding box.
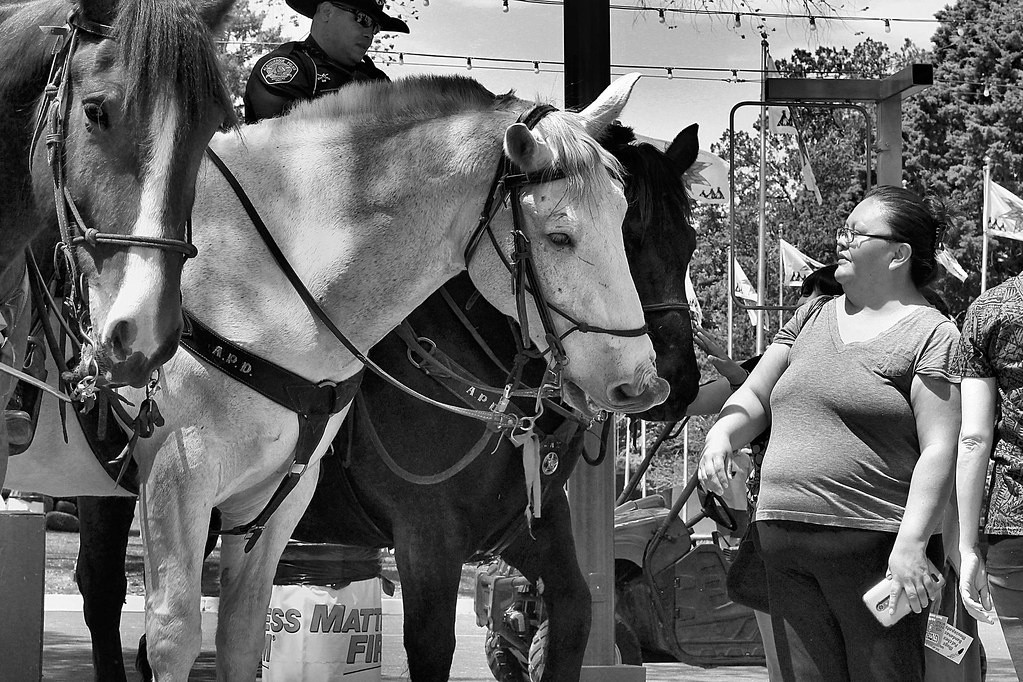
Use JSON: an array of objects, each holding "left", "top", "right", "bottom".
[{"left": 863, "top": 557, "right": 946, "bottom": 627}]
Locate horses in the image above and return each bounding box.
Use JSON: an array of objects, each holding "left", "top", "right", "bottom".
[
  {"left": 73, "top": 121, "right": 699, "bottom": 682},
  {"left": 0, "top": 73, "right": 670, "bottom": 682},
  {"left": 0, "top": 0, "right": 239, "bottom": 483}
]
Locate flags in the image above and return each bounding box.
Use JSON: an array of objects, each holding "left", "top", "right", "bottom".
[
  {"left": 734, "top": 258, "right": 769, "bottom": 331},
  {"left": 989, "top": 180, "right": 1023, "bottom": 242},
  {"left": 780, "top": 239, "right": 826, "bottom": 286},
  {"left": 685, "top": 268, "right": 703, "bottom": 327}
]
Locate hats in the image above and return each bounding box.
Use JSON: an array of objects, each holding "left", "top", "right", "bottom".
[{"left": 286, "top": 0, "right": 411, "bottom": 34}]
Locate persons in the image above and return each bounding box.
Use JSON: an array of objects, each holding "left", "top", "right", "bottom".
[
  {"left": 950, "top": 271, "right": 1023, "bottom": 682},
  {"left": 685, "top": 265, "right": 844, "bottom": 615},
  {"left": 243, "top": 0, "right": 410, "bottom": 126},
  {"left": 696, "top": 185, "right": 961, "bottom": 682}
]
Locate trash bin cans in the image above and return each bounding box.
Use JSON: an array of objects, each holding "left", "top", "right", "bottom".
[{"left": 262, "top": 543, "right": 384, "bottom": 682}]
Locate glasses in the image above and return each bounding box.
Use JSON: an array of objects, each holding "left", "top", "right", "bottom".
[
  {"left": 326, "top": 3, "right": 383, "bottom": 33},
  {"left": 835, "top": 227, "right": 908, "bottom": 246}
]
